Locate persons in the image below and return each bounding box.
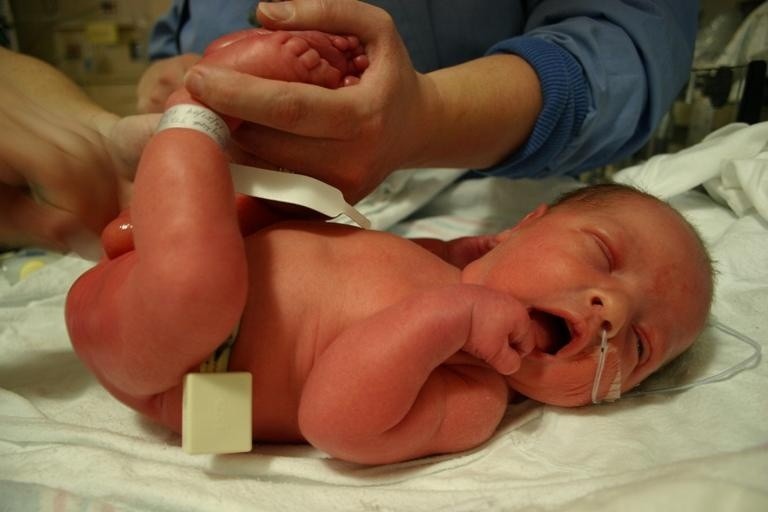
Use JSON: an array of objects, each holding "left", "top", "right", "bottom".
[
  {"left": 0, "top": 45, "right": 164, "bottom": 263},
  {"left": 137, "top": 0, "right": 700, "bottom": 220},
  {"left": 64, "top": 28, "right": 717, "bottom": 466}
]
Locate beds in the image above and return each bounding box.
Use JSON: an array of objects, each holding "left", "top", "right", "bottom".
[{"left": 0, "top": 119, "right": 767, "bottom": 512}]
